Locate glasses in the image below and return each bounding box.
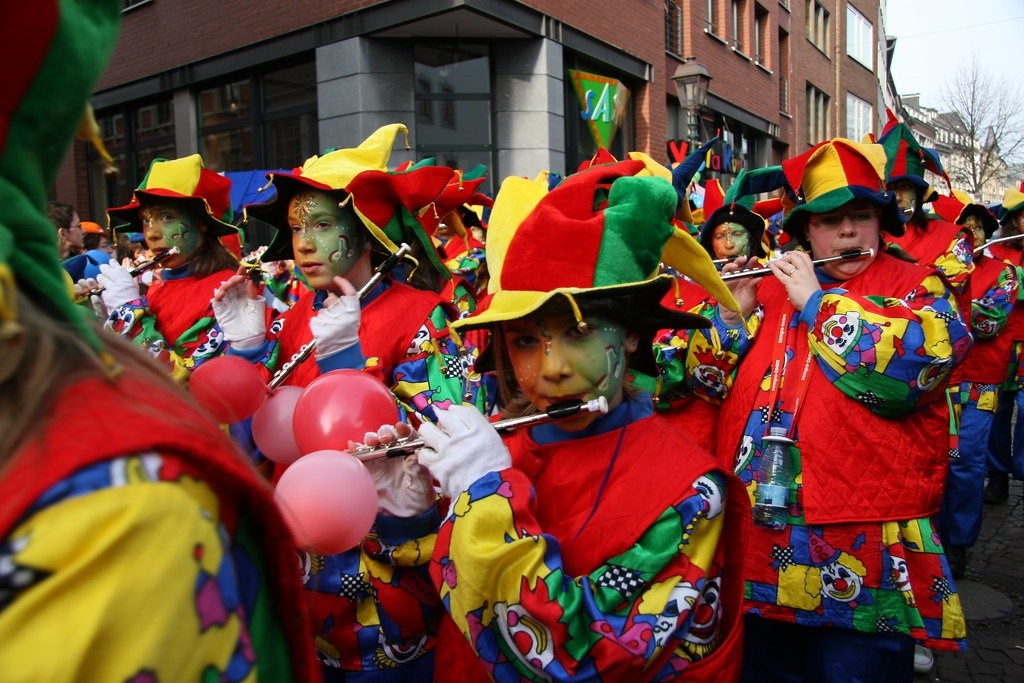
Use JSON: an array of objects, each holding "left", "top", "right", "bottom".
[{"left": 70, "top": 225, "right": 82, "bottom": 229}]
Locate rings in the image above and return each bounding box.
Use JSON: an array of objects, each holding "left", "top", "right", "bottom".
[{"left": 790, "top": 269, "right": 795, "bottom": 274}]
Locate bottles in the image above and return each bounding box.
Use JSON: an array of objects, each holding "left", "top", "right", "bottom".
[{"left": 752, "top": 427, "right": 791, "bottom": 530}]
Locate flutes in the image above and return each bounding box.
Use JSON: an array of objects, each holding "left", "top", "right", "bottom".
[
  {"left": 73, "top": 245, "right": 182, "bottom": 305},
  {"left": 269, "top": 242, "right": 413, "bottom": 388},
  {"left": 713, "top": 256, "right": 738, "bottom": 264},
  {"left": 987, "top": 232, "right": 1024, "bottom": 244},
  {"left": 349, "top": 397, "right": 611, "bottom": 461},
  {"left": 972, "top": 239, "right": 993, "bottom": 253},
  {"left": 720, "top": 247, "right": 876, "bottom": 281}
]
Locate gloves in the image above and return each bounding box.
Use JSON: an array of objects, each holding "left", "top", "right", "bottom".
[
  {"left": 73, "top": 277, "right": 105, "bottom": 314},
  {"left": 96, "top": 258, "right": 141, "bottom": 315}
]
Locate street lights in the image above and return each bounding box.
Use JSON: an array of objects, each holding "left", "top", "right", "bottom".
[{"left": 669, "top": 55, "right": 714, "bottom": 155}]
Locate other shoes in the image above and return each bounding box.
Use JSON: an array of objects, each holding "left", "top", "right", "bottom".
[
  {"left": 984, "top": 473, "right": 1010, "bottom": 502},
  {"left": 946, "top": 547, "right": 968, "bottom": 578},
  {"left": 913, "top": 645, "right": 935, "bottom": 674}
]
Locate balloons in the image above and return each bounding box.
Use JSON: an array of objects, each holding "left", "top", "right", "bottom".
[
  {"left": 273, "top": 450, "right": 377, "bottom": 553},
  {"left": 189, "top": 357, "right": 269, "bottom": 422},
  {"left": 253, "top": 386, "right": 305, "bottom": 466},
  {"left": 293, "top": 369, "right": 400, "bottom": 456}
]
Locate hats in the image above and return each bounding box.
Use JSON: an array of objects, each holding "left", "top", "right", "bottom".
[
  {"left": 988, "top": 181, "right": 1024, "bottom": 224},
  {"left": 0, "top": 0, "right": 126, "bottom": 378},
  {"left": 394, "top": 157, "right": 455, "bottom": 303},
  {"left": 79, "top": 222, "right": 109, "bottom": 238},
  {"left": 106, "top": 151, "right": 239, "bottom": 249},
  {"left": 242, "top": 122, "right": 419, "bottom": 283},
  {"left": 863, "top": 107, "right": 954, "bottom": 203},
  {"left": 928, "top": 189, "right": 1000, "bottom": 238},
  {"left": 434, "top": 162, "right": 494, "bottom": 210},
  {"left": 451, "top": 144, "right": 749, "bottom": 334},
  {"left": 672, "top": 168, "right": 766, "bottom": 259},
  {"left": 778, "top": 137, "right": 905, "bottom": 252}
]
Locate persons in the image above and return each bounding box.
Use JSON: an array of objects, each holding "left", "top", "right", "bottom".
[
  {"left": 0, "top": 0, "right": 318, "bottom": 683},
  {"left": 47, "top": 203, "right": 150, "bottom": 266},
  {"left": 75, "top": 155, "right": 264, "bottom": 393},
  {"left": 215, "top": 123, "right": 1024, "bottom": 682}
]
